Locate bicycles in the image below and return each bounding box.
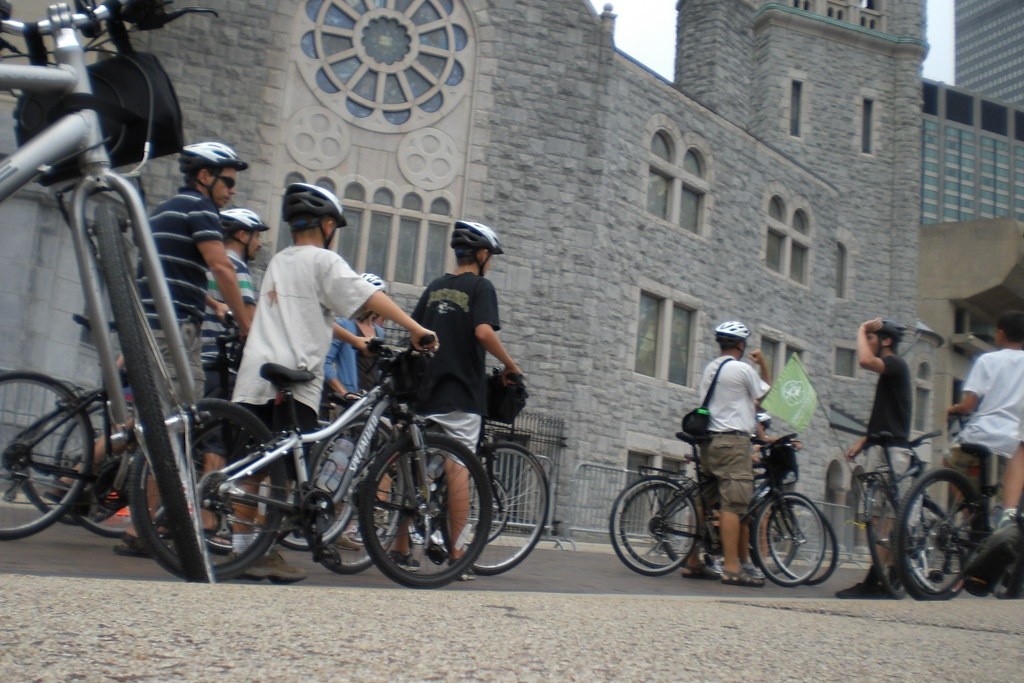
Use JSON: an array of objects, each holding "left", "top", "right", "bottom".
[
  {"left": 609, "top": 432, "right": 839, "bottom": 586},
  {"left": 894, "top": 412, "right": 1024, "bottom": 599},
  {"left": 0, "top": 298, "right": 551, "bottom": 586},
  {"left": 857, "top": 427, "right": 962, "bottom": 600},
  {"left": 0, "top": 0, "right": 224, "bottom": 582}
]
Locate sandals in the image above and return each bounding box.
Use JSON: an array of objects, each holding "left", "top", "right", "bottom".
[
  {"left": 681, "top": 563, "right": 721, "bottom": 581},
  {"left": 721, "top": 567, "right": 765, "bottom": 588},
  {"left": 42, "top": 481, "right": 91, "bottom": 517},
  {"left": 112, "top": 528, "right": 176, "bottom": 558}
]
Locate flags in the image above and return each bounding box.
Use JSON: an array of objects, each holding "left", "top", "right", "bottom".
[{"left": 761, "top": 352, "right": 820, "bottom": 434}]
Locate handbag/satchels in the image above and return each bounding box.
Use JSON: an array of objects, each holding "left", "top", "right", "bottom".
[
  {"left": 682, "top": 406, "right": 710, "bottom": 434},
  {"left": 770, "top": 443, "right": 799, "bottom": 486},
  {"left": 12, "top": 0, "right": 185, "bottom": 186},
  {"left": 393, "top": 350, "right": 432, "bottom": 402},
  {"left": 485, "top": 372, "right": 526, "bottom": 424}
]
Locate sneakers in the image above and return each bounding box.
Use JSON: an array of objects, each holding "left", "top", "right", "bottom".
[
  {"left": 228, "top": 547, "right": 306, "bottom": 585},
  {"left": 335, "top": 526, "right": 365, "bottom": 552},
  {"left": 449, "top": 558, "right": 478, "bottom": 580},
  {"left": 700, "top": 553, "right": 767, "bottom": 579},
  {"left": 835, "top": 565, "right": 898, "bottom": 600},
  {"left": 963, "top": 522, "right": 1018, "bottom": 576},
  {"left": 389, "top": 550, "right": 419, "bottom": 572}
]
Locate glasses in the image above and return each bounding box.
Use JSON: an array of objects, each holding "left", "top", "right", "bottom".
[{"left": 216, "top": 174, "right": 235, "bottom": 188}]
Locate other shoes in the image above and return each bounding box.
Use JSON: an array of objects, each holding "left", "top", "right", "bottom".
[{"left": 201, "top": 527, "right": 236, "bottom": 556}]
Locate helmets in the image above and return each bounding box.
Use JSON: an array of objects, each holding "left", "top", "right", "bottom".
[
  {"left": 218, "top": 208, "right": 269, "bottom": 237},
  {"left": 756, "top": 412, "right": 771, "bottom": 431},
  {"left": 451, "top": 220, "right": 505, "bottom": 255},
  {"left": 360, "top": 270, "right": 387, "bottom": 291},
  {"left": 179, "top": 142, "right": 248, "bottom": 177},
  {"left": 714, "top": 320, "right": 751, "bottom": 345},
  {"left": 871, "top": 320, "right": 910, "bottom": 342},
  {"left": 283, "top": 183, "right": 348, "bottom": 228}
]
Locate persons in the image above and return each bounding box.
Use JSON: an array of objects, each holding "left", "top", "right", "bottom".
[
  {"left": 681, "top": 319, "right": 802, "bottom": 587},
  {"left": 43, "top": 141, "right": 524, "bottom": 584},
  {"left": 942, "top": 310, "right": 1024, "bottom": 599},
  {"left": 835, "top": 317, "right": 914, "bottom": 599}
]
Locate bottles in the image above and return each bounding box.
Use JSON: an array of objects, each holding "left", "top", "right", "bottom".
[
  {"left": 858, "top": 480, "right": 877, "bottom": 520},
  {"left": 316, "top": 435, "right": 355, "bottom": 492},
  {"left": 425, "top": 455, "right": 444, "bottom": 485}
]
